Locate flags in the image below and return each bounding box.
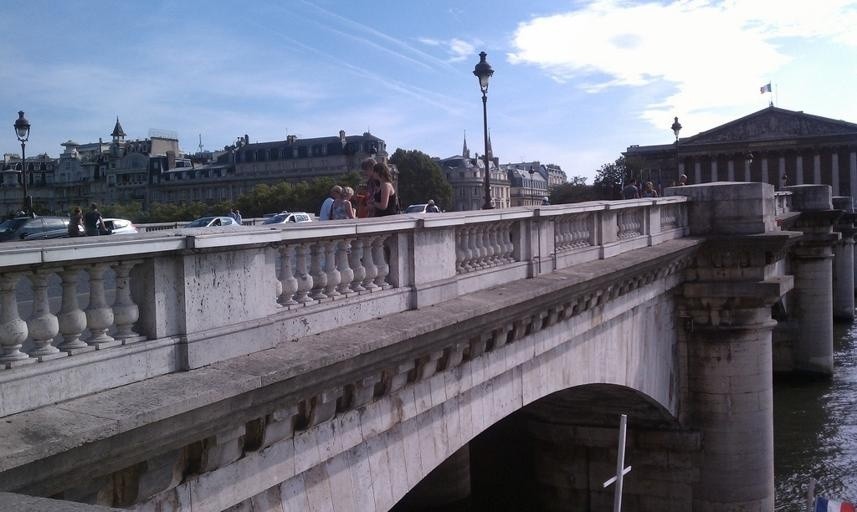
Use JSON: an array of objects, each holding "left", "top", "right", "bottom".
[
  {"left": 759, "top": 82, "right": 770, "bottom": 95},
  {"left": 812, "top": 493, "right": 857, "bottom": 512}
]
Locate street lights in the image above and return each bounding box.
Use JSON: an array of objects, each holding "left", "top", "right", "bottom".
[
  {"left": 742, "top": 148, "right": 754, "bottom": 181},
  {"left": 669, "top": 116, "right": 682, "bottom": 185},
  {"left": 11, "top": 110, "right": 31, "bottom": 211},
  {"left": 528, "top": 165, "right": 535, "bottom": 206},
  {"left": 470, "top": 51, "right": 495, "bottom": 208},
  {"left": 782, "top": 174, "right": 789, "bottom": 187}
]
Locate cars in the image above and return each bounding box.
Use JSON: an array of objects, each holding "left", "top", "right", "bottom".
[
  {"left": 405, "top": 203, "right": 440, "bottom": 213},
  {"left": 182, "top": 216, "right": 239, "bottom": 228},
  {"left": 95, "top": 217, "right": 138, "bottom": 235},
  {"left": 260, "top": 210, "right": 313, "bottom": 225}
]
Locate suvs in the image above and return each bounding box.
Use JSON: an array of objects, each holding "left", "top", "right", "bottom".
[{"left": 0, "top": 214, "right": 86, "bottom": 243}]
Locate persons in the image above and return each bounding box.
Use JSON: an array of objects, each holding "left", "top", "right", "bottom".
[
  {"left": 318, "top": 184, "right": 340, "bottom": 219},
  {"left": 618, "top": 178, "right": 640, "bottom": 199},
  {"left": 82, "top": 202, "right": 108, "bottom": 236},
  {"left": 425, "top": 199, "right": 440, "bottom": 213},
  {"left": 226, "top": 208, "right": 235, "bottom": 219},
  {"left": 676, "top": 174, "right": 687, "bottom": 186},
  {"left": 539, "top": 197, "right": 550, "bottom": 206},
  {"left": 329, "top": 187, "right": 354, "bottom": 219},
  {"left": 359, "top": 155, "right": 380, "bottom": 217},
  {"left": 233, "top": 210, "right": 242, "bottom": 225},
  {"left": 67, "top": 205, "right": 86, "bottom": 237},
  {"left": 368, "top": 163, "right": 400, "bottom": 215},
  {"left": 641, "top": 182, "right": 658, "bottom": 197}
]
